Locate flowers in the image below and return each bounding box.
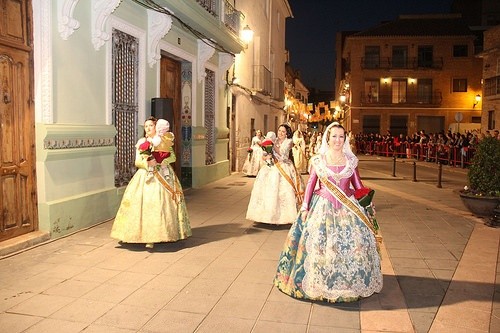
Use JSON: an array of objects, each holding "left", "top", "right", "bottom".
[
  {"left": 354, "top": 186, "right": 379, "bottom": 230},
  {"left": 136, "top": 136, "right": 161, "bottom": 172},
  {"left": 247, "top": 147, "right": 253, "bottom": 161},
  {"left": 262, "top": 140, "right": 274, "bottom": 166}
]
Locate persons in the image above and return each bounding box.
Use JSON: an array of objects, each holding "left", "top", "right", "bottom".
[
  {"left": 246, "top": 124, "right": 305, "bottom": 226},
  {"left": 242, "top": 124, "right": 323, "bottom": 176},
  {"left": 110, "top": 116, "right": 192, "bottom": 249},
  {"left": 144, "top": 119, "right": 175, "bottom": 185},
  {"left": 274, "top": 122, "right": 383, "bottom": 304},
  {"left": 349, "top": 128, "right": 500, "bottom": 168}
]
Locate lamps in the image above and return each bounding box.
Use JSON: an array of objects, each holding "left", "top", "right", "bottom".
[
  {"left": 242, "top": 25, "right": 252, "bottom": 49},
  {"left": 473, "top": 94, "right": 482, "bottom": 109}
]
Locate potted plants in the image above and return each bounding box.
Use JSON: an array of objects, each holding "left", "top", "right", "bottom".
[{"left": 458, "top": 135, "right": 500, "bottom": 217}]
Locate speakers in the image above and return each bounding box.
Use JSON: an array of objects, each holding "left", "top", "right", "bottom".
[{"left": 151, "top": 98, "right": 173, "bottom": 132}]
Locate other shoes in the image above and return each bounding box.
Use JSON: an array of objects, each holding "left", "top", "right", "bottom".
[
  {"left": 165, "top": 176, "right": 171, "bottom": 181},
  {"left": 145, "top": 173, "right": 153, "bottom": 183},
  {"left": 146, "top": 243, "right": 153, "bottom": 248}
]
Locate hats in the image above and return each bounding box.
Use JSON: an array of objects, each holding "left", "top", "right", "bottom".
[{"left": 155, "top": 118, "right": 169, "bottom": 134}]
